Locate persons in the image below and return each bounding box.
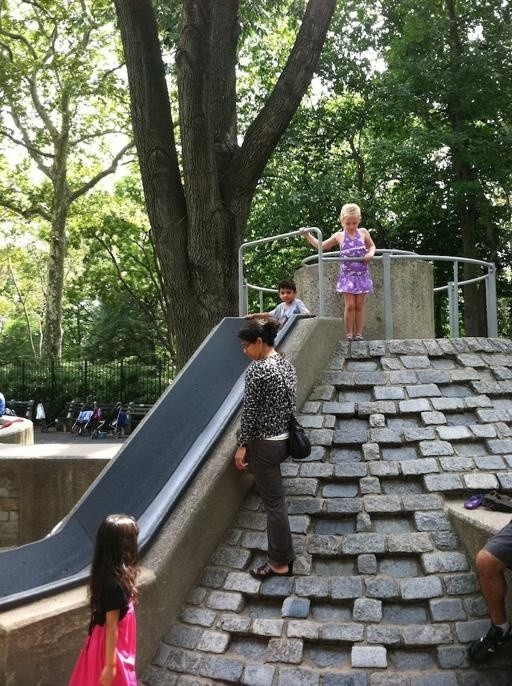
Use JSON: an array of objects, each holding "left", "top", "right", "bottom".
[
  {"left": 0, "top": 392, "right": 6, "bottom": 417},
  {"left": 299, "top": 202, "right": 377, "bottom": 342},
  {"left": 92, "top": 401, "right": 101, "bottom": 421},
  {"left": 235, "top": 318, "right": 299, "bottom": 578},
  {"left": 245, "top": 279, "right": 312, "bottom": 327},
  {"left": 68, "top": 512, "right": 140, "bottom": 686},
  {"left": 468, "top": 520, "right": 511, "bottom": 664}
]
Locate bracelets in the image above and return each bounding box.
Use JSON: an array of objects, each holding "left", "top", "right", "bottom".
[{"left": 105, "top": 662, "right": 116, "bottom": 668}]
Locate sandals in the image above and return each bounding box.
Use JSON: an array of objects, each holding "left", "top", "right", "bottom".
[
  {"left": 484, "top": 490, "right": 512, "bottom": 514},
  {"left": 464, "top": 493, "right": 483, "bottom": 509}
]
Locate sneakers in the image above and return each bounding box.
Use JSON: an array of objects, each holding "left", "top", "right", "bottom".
[
  {"left": 346, "top": 334, "right": 364, "bottom": 342},
  {"left": 468, "top": 623, "right": 512, "bottom": 664}
]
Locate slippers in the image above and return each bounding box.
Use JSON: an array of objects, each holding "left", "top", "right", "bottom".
[{"left": 249, "top": 562, "right": 290, "bottom": 579}]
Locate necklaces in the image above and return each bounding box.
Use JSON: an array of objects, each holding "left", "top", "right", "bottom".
[{"left": 263, "top": 351, "right": 273, "bottom": 356}]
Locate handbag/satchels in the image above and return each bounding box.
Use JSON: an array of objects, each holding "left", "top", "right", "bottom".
[{"left": 289, "top": 414, "right": 311, "bottom": 459}]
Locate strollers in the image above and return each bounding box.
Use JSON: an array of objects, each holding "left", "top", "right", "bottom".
[
  {"left": 91, "top": 401, "right": 129, "bottom": 440},
  {"left": 71, "top": 399, "right": 102, "bottom": 436}
]
